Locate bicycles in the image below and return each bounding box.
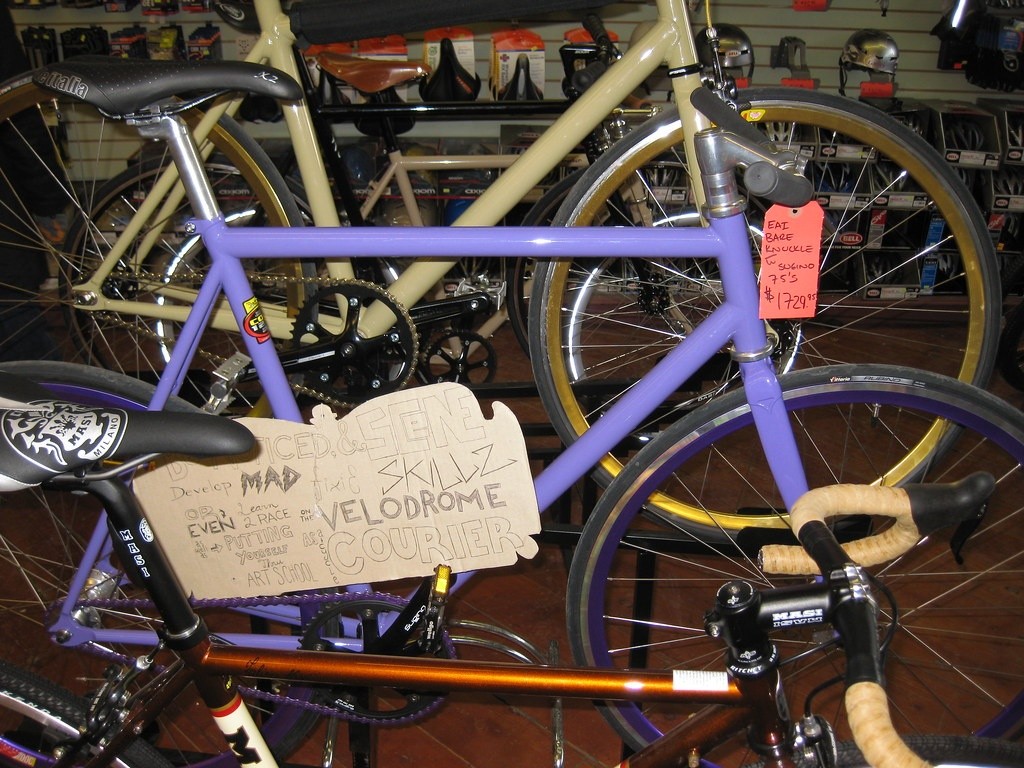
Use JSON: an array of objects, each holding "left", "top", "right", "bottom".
[
  {"left": 0, "top": 0, "right": 1005, "bottom": 543},
  {"left": 0, "top": 52, "right": 1024, "bottom": 768},
  {"left": 0, "top": 362, "right": 1024, "bottom": 768}
]
